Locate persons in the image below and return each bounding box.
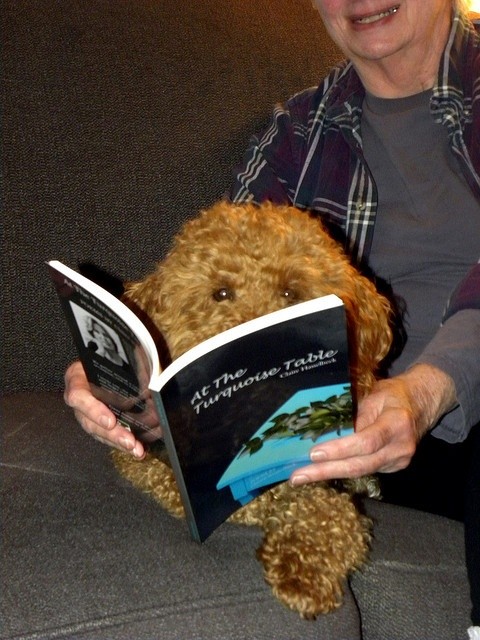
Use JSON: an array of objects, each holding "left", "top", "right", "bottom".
[
  {"left": 84, "top": 315, "right": 126, "bottom": 369},
  {"left": 65, "top": 0, "right": 479, "bottom": 640}
]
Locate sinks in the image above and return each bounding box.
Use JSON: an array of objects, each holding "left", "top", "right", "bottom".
[{"left": 2, "top": 0, "right": 480, "bottom": 639}]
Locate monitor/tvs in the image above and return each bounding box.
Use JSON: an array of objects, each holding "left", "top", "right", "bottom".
[{"left": 46, "top": 259, "right": 357, "bottom": 545}]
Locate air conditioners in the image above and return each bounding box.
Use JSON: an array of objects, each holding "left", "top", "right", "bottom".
[{"left": 100, "top": 194, "right": 396, "bottom": 621}]
